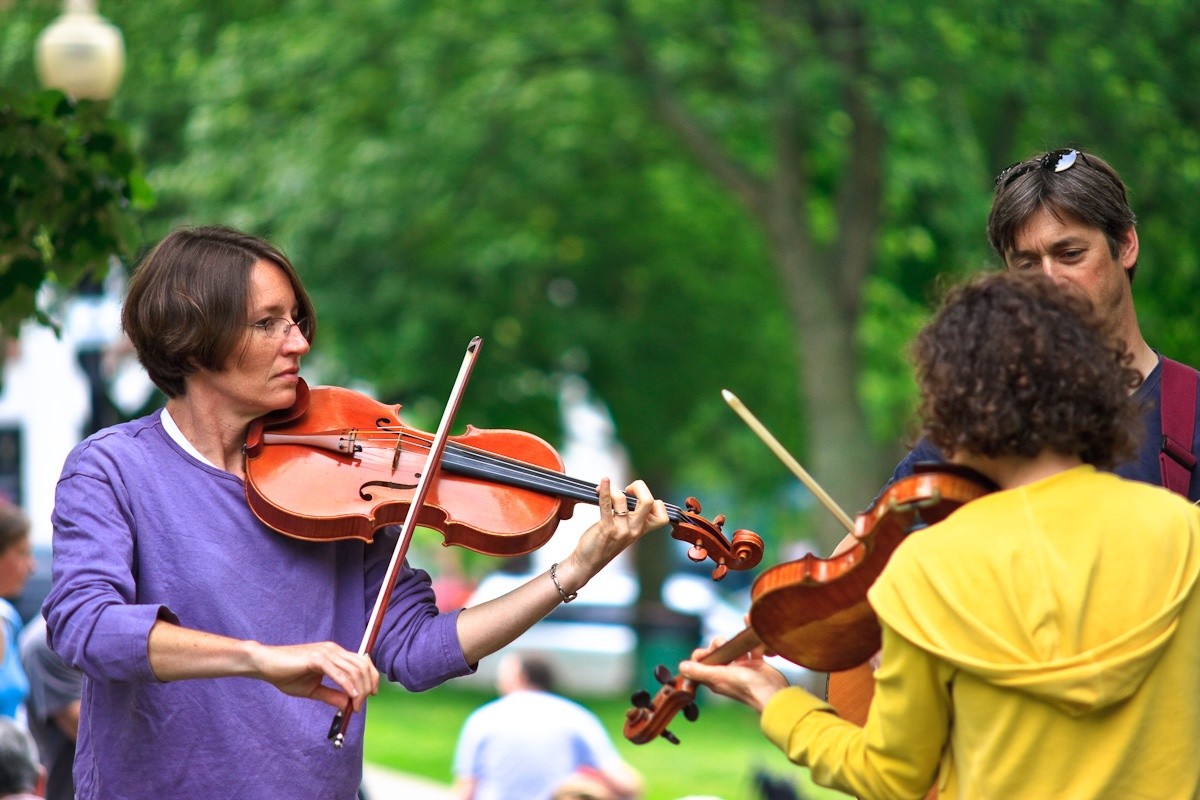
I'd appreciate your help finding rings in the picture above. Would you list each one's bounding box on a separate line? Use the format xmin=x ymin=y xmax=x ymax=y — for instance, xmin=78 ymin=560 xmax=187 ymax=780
xmin=613 ymin=509 xmax=630 ymax=516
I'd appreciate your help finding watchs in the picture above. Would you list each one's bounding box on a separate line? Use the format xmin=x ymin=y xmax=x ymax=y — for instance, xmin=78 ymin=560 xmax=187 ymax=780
xmin=551 ymin=563 xmax=577 ymax=603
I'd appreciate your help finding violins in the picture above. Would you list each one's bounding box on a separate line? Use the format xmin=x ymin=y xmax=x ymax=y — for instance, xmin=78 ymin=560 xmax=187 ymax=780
xmin=824 ymin=648 xmax=878 ymax=730
xmin=240 ymin=386 xmax=765 ymax=582
xmin=620 ymin=471 xmax=1003 ymax=747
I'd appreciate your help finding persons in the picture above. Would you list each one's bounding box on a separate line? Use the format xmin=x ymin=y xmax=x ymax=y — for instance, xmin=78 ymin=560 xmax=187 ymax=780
xmin=853 ymin=148 xmax=1200 ymax=505
xmin=0 ymin=498 xmax=83 ymax=800
xmin=679 ymin=266 xmax=1200 ymax=799
xmin=451 ymin=648 xmax=644 ymax=800
xmin=40 ymin=225 xmax=671 ymax=800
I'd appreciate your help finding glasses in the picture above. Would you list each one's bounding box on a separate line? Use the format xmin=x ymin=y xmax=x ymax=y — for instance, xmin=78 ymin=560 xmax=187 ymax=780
xmin=994 ymin=147 xmax=1093 ymax=187
xmin=245 ymin=318 xmax=312 ymax=339
